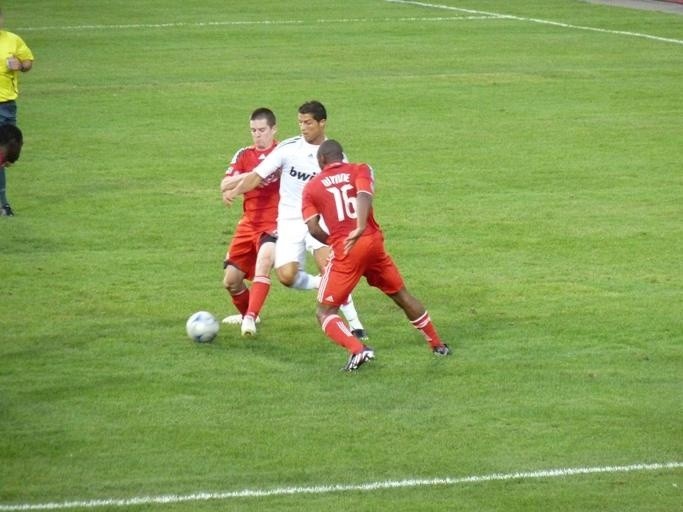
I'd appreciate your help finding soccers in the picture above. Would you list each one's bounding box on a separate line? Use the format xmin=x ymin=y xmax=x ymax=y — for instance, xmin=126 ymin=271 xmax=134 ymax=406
xmin=187 ymin=311 xmax=219 ymax=342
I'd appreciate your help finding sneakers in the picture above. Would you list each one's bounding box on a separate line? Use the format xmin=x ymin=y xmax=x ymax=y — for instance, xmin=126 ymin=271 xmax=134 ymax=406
xmin=431 ymin=343 xmax=452 ymax=359
xmin=221 ymin=313 xmax=261 ymax=325
xmin=0 ymin=203 xmax=14 ymax=217
xmin=337 ymin=344 xmax=375 ymax=374
xmin=341 ymin=329 xmax=369 ymax=347
xmin=239 ymin=315 xmax=256 ymax=337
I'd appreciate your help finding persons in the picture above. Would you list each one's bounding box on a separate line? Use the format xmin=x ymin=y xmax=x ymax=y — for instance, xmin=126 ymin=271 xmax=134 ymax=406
xmin=0 ymin=124 xmax=23 ymax=170
xmin=301 ymin=139 xmax=451 ymax=374
xmin=221 ymin=99 xmax=368 ymax=343
xmin=0 ymin=29 xmax=34 ymax=217
xmin=221 ymin=108 xmax=281 ymax=337
